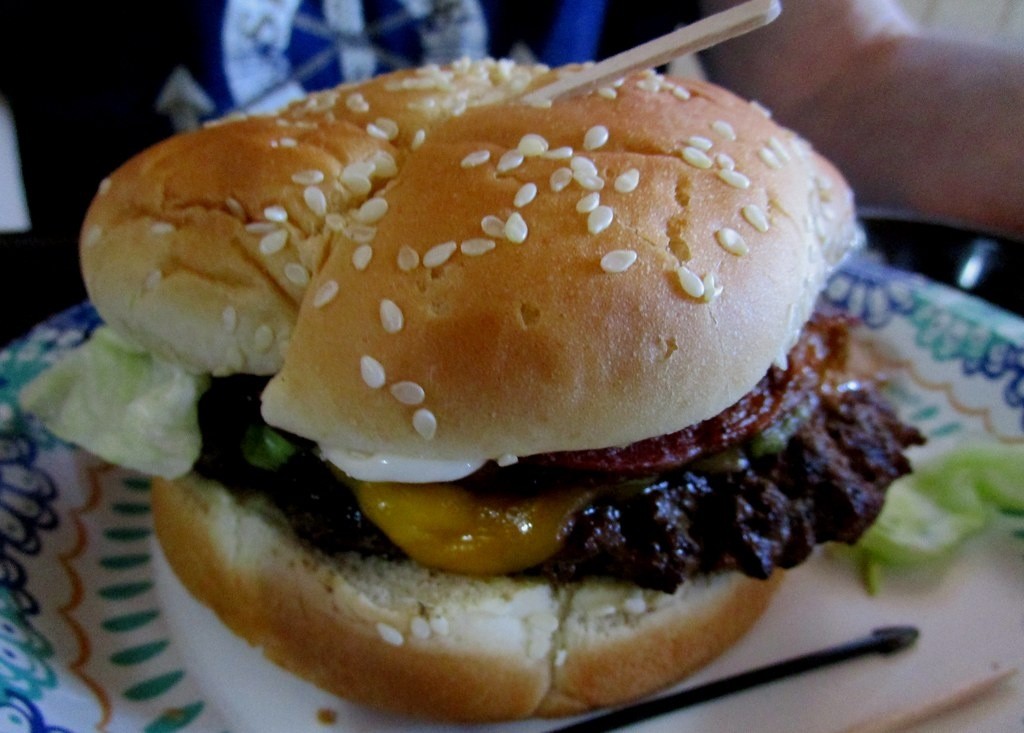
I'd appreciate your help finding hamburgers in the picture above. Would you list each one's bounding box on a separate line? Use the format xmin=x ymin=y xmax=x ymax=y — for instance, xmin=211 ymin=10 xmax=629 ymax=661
xmin=13 ymin=54 xmax=1024 ymax=725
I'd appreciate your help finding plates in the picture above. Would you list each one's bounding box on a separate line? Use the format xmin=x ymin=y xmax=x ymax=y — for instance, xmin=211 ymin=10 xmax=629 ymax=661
xmin=2 ymin=254 xmax=1024 ymax=733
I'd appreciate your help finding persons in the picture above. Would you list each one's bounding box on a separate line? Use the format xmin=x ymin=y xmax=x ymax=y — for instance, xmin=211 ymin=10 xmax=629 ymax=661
xmin=3 ymin=0 xmax=1023 ymax=343
